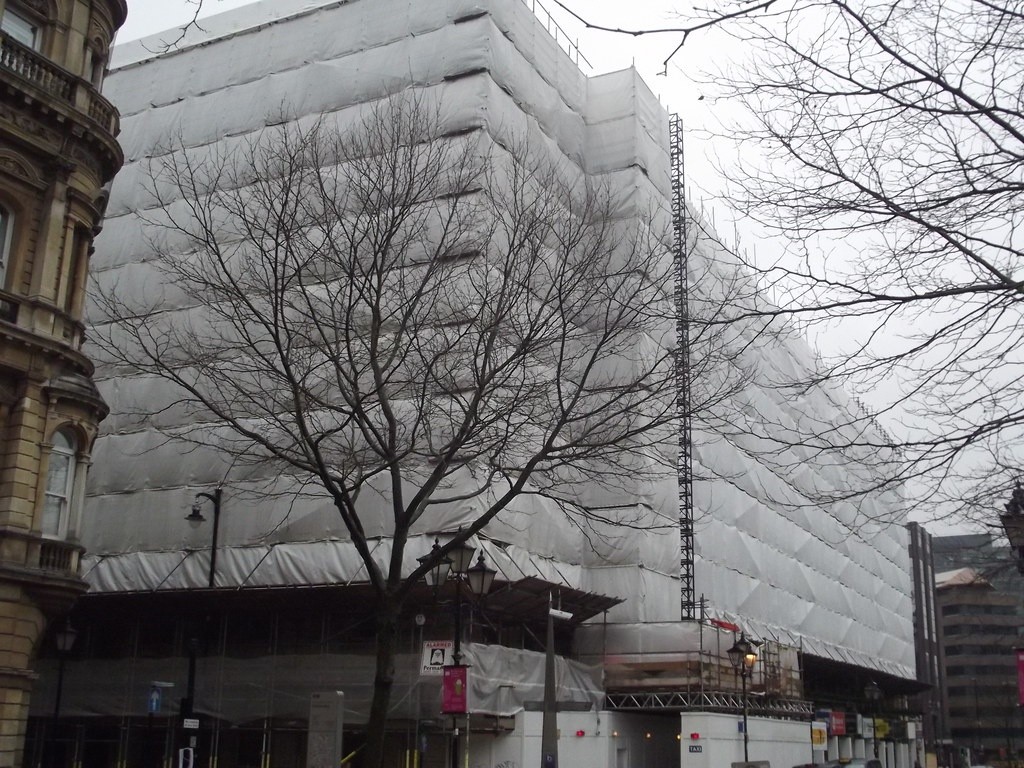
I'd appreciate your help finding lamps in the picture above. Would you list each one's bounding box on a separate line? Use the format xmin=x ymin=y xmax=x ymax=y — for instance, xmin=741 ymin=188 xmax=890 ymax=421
xmin=183 ymin=493 xmax=219 ymax=528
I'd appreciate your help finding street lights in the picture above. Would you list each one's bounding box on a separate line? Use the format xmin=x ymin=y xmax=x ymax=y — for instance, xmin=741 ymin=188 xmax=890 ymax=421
xmin=417 ymin=524 xmax=498 ymax=768
xmin=726 ymin=631 xmax=759 ymax=762
xmin=166 ymin=486 xmax=224 ymax=768
xmin=864 ymin=675 xmax=882 ymax=759
